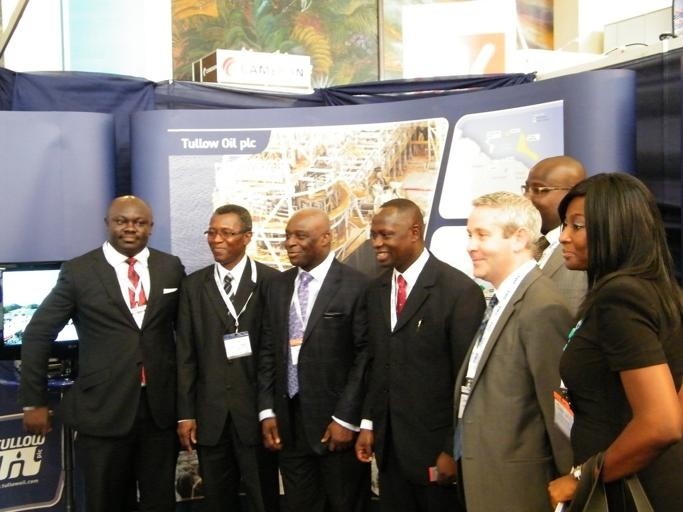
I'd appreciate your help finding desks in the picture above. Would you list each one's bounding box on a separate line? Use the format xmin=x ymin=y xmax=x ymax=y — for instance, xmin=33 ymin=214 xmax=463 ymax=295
xmin=0 ymin=361 xmax=82 ymax=510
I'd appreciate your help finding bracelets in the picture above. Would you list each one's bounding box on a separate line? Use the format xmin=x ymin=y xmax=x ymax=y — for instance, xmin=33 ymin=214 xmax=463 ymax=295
xmin=574 ymin=466 xmax=583 ymax=481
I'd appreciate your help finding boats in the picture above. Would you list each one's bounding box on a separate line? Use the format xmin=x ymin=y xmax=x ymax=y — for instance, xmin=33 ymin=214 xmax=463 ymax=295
xmin=213 ymin=120 xmax=438 ymax=273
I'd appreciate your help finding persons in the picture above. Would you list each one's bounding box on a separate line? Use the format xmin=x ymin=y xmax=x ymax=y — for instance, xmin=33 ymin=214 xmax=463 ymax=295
xmin=520 ymin=156 xmax=588 ymax=319
xmin=175 ymin=204 xmax=283 ymax=512
xmin=547 ymin=170 xmax=682 ymax=511
xmin=354 ymin=200 xmax=486 ymax=511
xmin=20 ymin=196 xmax=187 ymax=511
xmin=252 ymin=208 xmax=375 ymax=512
xmin=453 ymin=192 xmax=576 ymax=512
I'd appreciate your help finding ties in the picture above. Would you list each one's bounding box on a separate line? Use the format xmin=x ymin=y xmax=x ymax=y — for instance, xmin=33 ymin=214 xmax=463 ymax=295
xmin=470 ymin=294 xmax=501 ymax=352
xmin=126 ymin=256 xmax=147 ymax=386
xmin=396 ymin=275 xmax=408 ymax=321
xmin=222 ymin=275 xmax=238 ymax=322
xmin=286 ymin=271 xmax=313 ymax=399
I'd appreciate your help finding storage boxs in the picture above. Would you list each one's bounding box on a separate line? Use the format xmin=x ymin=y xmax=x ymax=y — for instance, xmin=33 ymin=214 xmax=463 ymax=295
xmin=188 ymin=47 xmax=314 ymax=94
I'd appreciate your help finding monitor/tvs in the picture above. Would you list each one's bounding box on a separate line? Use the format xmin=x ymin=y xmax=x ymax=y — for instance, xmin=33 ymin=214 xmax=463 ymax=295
xmin=0 ymin=260 xmax=79 ymax=360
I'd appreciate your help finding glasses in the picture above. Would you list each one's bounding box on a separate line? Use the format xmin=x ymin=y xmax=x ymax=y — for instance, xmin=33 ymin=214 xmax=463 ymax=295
xmin=522 ymin=183 xmax=572 ymax=198
xmin=204 ymin=226 xmax=244 ymax=239
